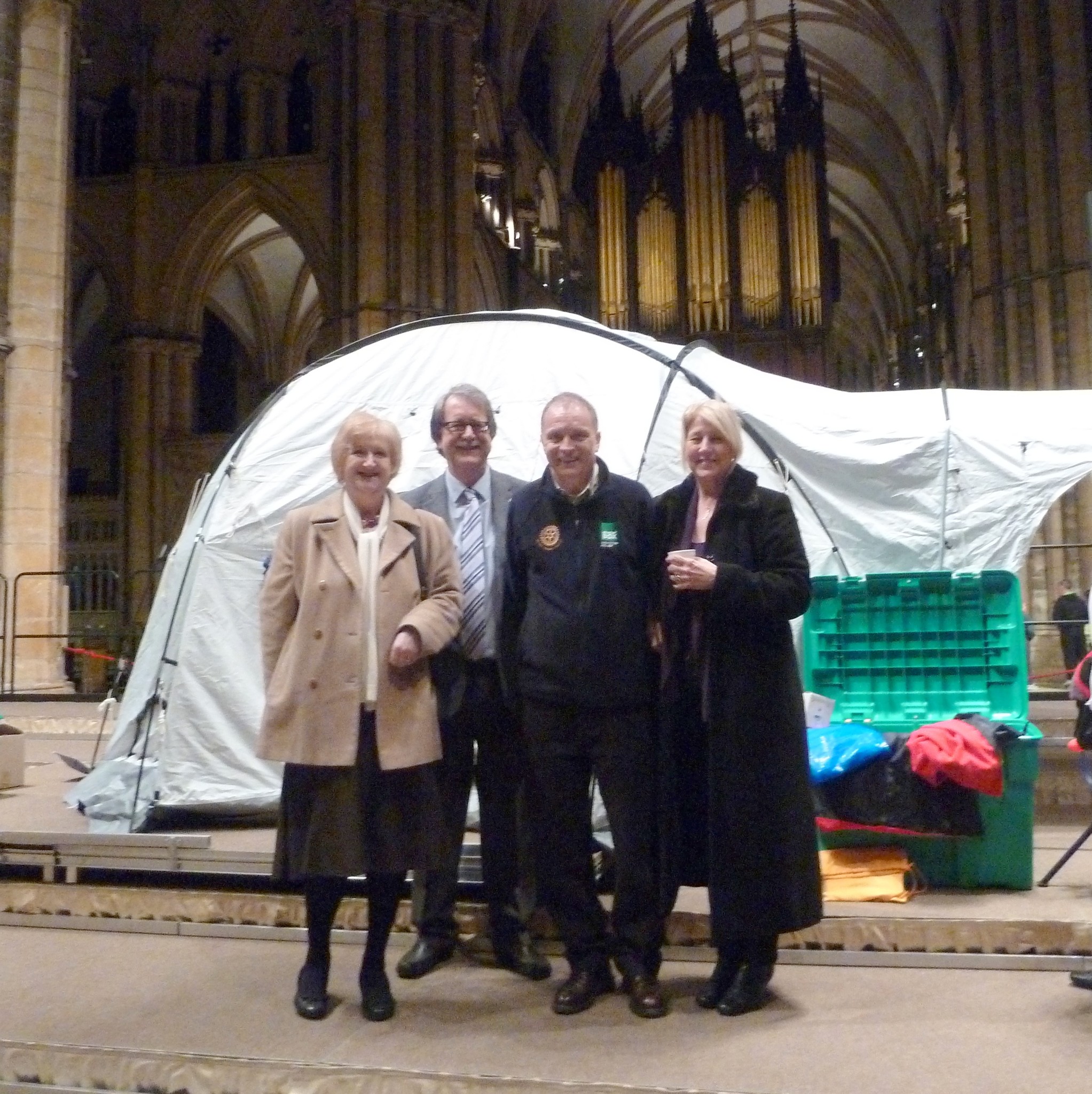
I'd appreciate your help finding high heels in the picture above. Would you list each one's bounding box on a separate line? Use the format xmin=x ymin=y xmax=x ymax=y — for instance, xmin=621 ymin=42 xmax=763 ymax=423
xmin=697 ymin=955 xmax=741 ymax=1008
xmin=719 ymin=960 xmax=774 ymax=1015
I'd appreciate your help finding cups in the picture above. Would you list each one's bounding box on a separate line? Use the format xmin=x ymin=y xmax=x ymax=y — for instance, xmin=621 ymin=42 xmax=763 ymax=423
xmin=667 ymin=549 xmax=696 ymax=585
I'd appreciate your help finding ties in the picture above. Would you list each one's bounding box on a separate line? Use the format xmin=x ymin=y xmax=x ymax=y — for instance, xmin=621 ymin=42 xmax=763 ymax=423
xmin=463 ymin=487 xmax=487 ymax=662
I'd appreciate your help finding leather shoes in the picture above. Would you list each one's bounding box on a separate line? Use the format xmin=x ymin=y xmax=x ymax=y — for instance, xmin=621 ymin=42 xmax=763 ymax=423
xmin=622 ymin=969 xmax=665 ymax=1019
xmin=396 ymin=931 xmax=454 ymax=977
xmin=295 ymin=957 xmax=328 ymax=1020
xmin=491 ymin=933 xmax=552 ymax=980
xmin=359 ymin=968 xmax=394 ymax=1023
xmin=553 ymin=964 xmax=612 ymax=1014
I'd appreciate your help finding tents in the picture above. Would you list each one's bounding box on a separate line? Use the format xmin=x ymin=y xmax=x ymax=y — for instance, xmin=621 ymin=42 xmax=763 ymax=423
xmin=104 ymin=310 xmax=1092 ymax=824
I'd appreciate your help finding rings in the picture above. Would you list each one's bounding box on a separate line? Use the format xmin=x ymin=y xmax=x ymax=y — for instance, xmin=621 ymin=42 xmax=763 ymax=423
xmin=676 ymin=575 xmax=681 ymax=582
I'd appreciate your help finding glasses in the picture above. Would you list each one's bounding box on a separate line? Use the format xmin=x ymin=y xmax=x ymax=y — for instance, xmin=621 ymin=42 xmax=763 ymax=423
xmin=442 ymin=421 xmax=491 ymax=433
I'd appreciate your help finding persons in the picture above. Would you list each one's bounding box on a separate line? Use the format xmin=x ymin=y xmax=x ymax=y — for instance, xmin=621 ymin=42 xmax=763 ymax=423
xmin=496 ymin=393 xmax=669 ymax=1017
xmin=1053 ymin=579 xmax=1089 ymax=685
xmin=1068 ymin=650 xmax=1092 ymax=787
xmin=396 ymin=383 xmax=552 ymax=979
xmin=650 ymin=399 xmax=823 ymax=1013
xmin=255 ymin=412 xmax=464 ymax=1023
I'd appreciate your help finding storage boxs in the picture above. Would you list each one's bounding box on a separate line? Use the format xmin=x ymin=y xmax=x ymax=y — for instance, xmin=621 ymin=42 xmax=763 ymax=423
xmin=794 ymin=567 xmax=1045 ymax=893
xmin=0 ymin=723 xmax=26 ymax=790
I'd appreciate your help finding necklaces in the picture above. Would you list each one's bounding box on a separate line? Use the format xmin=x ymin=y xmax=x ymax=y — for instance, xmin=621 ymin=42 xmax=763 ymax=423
xmin=701 ymin=509 xmax=709 ymax=520
